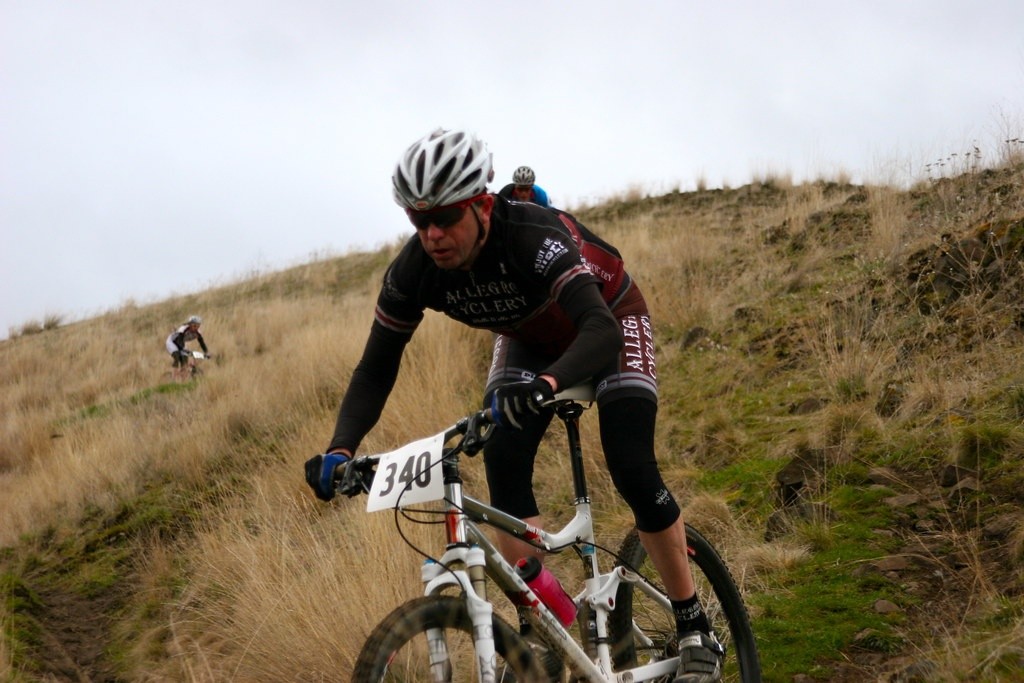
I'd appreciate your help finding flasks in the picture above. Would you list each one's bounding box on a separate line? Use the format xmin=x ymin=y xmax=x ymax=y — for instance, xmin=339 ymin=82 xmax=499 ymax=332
xmin=514 ymin=554 xmax=580 ymax=628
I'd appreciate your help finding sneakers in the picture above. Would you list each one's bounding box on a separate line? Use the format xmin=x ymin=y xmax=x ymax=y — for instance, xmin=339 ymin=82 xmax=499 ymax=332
xmin=672 ymin=629 xmax=726 ymax=683
xmin=499 ymin=641 xmax=559 ymax=683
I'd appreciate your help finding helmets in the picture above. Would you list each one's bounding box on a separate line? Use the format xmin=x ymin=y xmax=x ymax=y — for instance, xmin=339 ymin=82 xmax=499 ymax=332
xmin=391 ymin=126 xmax=494 ymax=210
xmin=512 ymin=165 xmax=535 ymax=185
xmin=189 ymin=316 xmax=201 ymax=323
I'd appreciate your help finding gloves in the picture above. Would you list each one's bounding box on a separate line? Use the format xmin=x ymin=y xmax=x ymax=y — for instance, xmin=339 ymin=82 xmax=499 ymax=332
xmin=303 ymin=451 xmax=350 ymax=501
xmin=493 ymin=378 xmax=556 ymax=431
xmin=205 ymin=353 xmax=211 ymax=359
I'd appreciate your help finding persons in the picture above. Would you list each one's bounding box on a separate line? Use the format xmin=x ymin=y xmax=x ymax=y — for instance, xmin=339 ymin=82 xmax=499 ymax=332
xmin=303 ymin=127 xmax=726 ymax=683
xmin=498 ymin=166 xmax=546 ymax=210
xmin=166 ymin=316 xmax=210 ymax=380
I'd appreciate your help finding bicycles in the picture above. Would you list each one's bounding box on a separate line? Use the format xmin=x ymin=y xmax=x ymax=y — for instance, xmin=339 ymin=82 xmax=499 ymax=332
xmin=158 ymin=349 xmax=211 ymax=386
xmin=328 ymin=380 xmax=763 ymax=683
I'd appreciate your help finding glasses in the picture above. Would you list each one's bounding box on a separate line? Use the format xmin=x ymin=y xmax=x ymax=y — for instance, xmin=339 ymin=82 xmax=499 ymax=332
xmin=516 ymin=187 xmax=531 ymax=192
xmin=406 ymin=196 xmax=486 ymax=230
